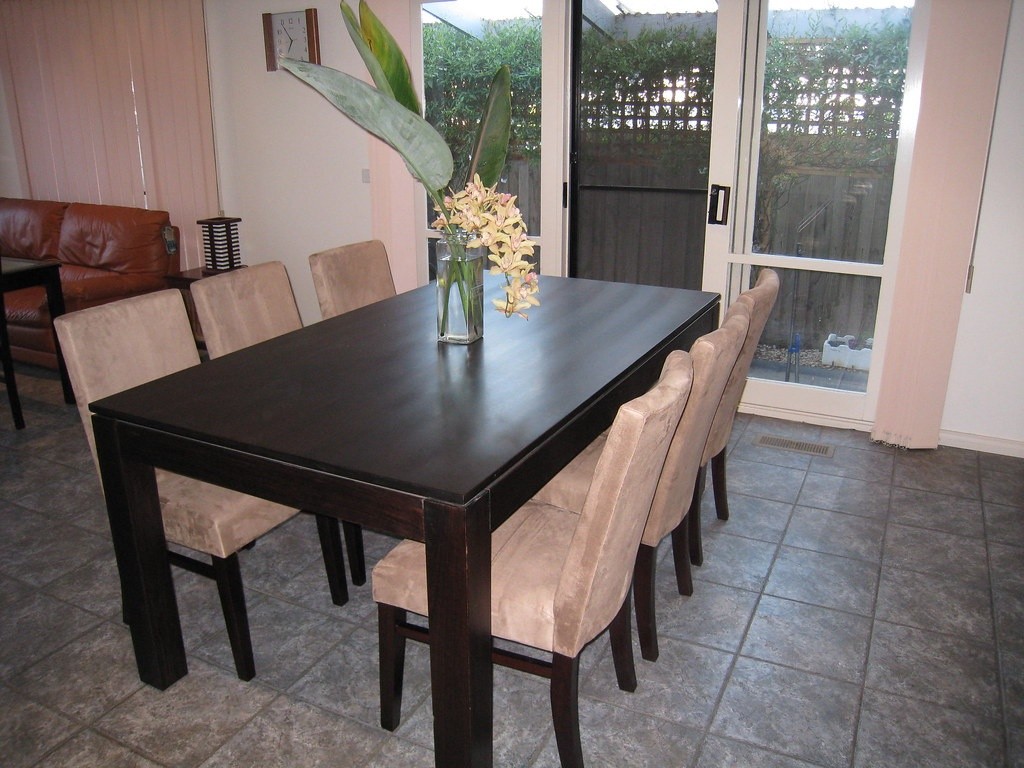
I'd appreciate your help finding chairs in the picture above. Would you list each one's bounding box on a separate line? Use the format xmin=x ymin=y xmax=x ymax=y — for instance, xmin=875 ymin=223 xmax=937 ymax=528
xmin=531 ymin=302 xmax=751 ymax=661
xmin=189 ymin=261 xmax=367 ymax=587
xmin=685 ymin=267 xmax=781 ymax=567
xmin=53 ymin=288 xmax=347 ymax=681
xmin=373 ymin=350 xmax=693 ymax=768
xmin=309 ymin=239 xmax=397 ymax=323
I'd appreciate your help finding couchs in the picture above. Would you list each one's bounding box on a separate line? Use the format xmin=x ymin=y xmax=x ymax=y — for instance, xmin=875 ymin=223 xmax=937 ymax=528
xmin=0 ymin=197 xmax=181 ymax=374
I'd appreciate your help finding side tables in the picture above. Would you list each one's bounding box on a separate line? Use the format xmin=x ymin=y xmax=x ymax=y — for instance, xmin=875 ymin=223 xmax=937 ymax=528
xmin=162 ymin=265 xmax=247 ymax=352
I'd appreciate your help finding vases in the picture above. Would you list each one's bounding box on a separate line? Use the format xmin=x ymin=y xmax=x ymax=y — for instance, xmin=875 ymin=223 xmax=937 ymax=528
xmin=434 ymin=230 xmax=485 ymax=343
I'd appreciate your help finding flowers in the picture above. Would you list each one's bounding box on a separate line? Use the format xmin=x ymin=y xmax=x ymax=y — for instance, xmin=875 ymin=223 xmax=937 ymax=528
xmin=431 ymin=175 xmax=540 ymax=322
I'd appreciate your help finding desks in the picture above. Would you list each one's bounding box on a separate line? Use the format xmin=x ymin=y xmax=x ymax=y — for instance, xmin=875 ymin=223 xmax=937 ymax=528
xmin=89 ymin=268 xmax=721 ymax=768
xmin=0 ymin=256 xmax=76 ymax=430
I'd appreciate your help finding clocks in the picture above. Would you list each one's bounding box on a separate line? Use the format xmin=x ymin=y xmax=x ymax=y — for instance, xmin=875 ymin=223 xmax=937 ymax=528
xmin=262 ymin=7 xmax=321 ymax=71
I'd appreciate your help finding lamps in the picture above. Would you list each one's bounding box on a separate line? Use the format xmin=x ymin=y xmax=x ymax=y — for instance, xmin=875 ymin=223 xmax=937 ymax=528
xmin=783 ymin=169 xmax=874 ymax=383
xmin=196 ymin=216 xmax=249 ymax=276
